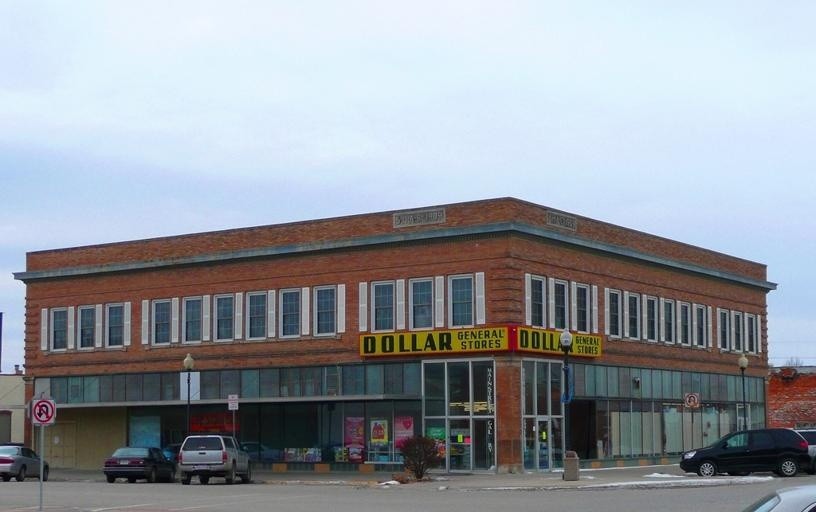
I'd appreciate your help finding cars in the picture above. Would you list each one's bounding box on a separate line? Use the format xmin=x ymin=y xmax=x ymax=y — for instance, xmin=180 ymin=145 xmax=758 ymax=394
xmin=680 ymin=428 xmax=816 ymax=477
xmin=741 ymin=485 xmax=816 ymax=512
xmin=239 ymin=442 xmax=283 ymax=461
xmin=0 ymin=442 xmax=49 ymax=482
xmin=177 ymin=436 xmax=252 ymax=484
xmin=103 ymin=447 xmax=176 ymax=483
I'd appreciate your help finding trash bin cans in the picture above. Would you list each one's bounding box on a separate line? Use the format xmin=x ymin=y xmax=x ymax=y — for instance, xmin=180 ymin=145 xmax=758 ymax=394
xmin=563 ymin=451 xmax=580 ymax=481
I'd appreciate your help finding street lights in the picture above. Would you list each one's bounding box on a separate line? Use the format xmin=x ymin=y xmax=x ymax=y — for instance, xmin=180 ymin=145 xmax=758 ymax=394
xmin=738 ymin=354 xmax=748 ymax=430
xmin=558 ymin=328 xmax=573 ymax=401
xmin=183 ymin=353 xmax=194 ymax=437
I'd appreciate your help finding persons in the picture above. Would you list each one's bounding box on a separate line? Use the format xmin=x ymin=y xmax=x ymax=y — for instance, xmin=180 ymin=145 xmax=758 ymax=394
xmin=437 ymin=440 xmax=446 ymax=468
